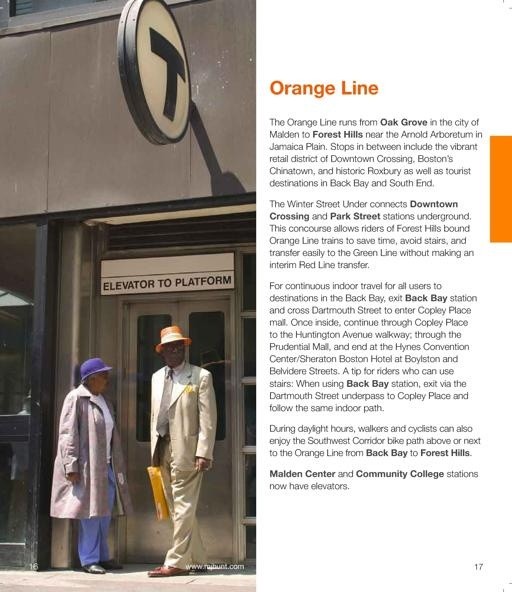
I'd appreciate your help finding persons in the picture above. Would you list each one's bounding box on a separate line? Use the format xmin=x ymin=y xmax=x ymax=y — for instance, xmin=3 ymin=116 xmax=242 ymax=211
xmin=50 ymin=358 xmax=137 ymax=573
xmin=146 ymin=325 xmax=217 ymax=577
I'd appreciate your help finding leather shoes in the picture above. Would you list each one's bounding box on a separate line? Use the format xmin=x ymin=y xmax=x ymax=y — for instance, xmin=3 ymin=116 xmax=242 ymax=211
xmin=190 ymin=566 xmax=209 ymax=573
xmin=82 ymin=563 xmax=107 ymax=575
xmin=100 ymin=559 xmax=124 ymax=570
xmin=147 ymin=564 xmax=191 ymax=577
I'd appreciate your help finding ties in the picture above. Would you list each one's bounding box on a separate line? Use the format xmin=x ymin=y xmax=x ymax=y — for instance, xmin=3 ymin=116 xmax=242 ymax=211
xmin=157 ymin=368 xmax=175 ymax=438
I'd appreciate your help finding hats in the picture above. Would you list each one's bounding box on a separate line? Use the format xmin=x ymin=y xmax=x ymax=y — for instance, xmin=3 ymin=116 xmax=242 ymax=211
xmin=154 ymin=324 xmax=192 ymax=354
xmin=79 ymin=356 xmax=114 ymax=382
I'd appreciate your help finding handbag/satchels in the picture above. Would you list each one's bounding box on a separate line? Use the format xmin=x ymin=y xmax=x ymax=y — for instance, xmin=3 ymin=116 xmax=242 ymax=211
xmin=146 ymin=465 xmax=171 ymax=523
